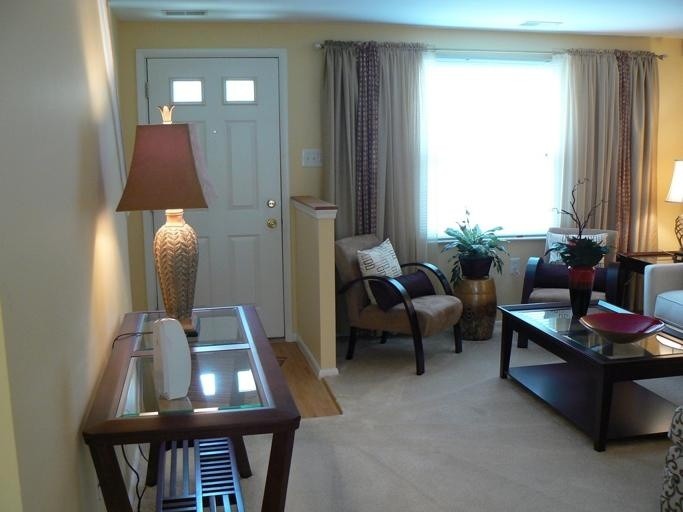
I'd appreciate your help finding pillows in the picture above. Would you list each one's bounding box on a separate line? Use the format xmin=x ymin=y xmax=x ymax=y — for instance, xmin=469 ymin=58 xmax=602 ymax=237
xmin=534 ymin=265 xmax=608 ymax=292
xmin=547 ymin=233 xmax=608 ymax=269
xmin=368 ymin=269 xmax=436 ymax=312
xmin=356 ymin=238 xmax=404 ymax=306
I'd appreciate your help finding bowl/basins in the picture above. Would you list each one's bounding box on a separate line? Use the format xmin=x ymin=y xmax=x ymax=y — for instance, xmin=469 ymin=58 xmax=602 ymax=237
xmin=580 ymin=312 xmax=666 ymax=345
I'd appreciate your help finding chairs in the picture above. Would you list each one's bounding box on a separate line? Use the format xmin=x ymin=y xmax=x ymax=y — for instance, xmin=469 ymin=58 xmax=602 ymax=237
xmin=335 ymin=233 xmax=463 ymax=375
xmin=517 ymin=226 xmax=627 ymax=348
xmin=643 ymin=262 xmax=683 ymax=334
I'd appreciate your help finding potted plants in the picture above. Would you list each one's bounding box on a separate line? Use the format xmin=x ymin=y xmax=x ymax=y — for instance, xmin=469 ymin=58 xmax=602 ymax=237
xmin=440 ymin=219 xmax=511 ymax=290
xmin=543 ymin=177 xmax=611 ymax=318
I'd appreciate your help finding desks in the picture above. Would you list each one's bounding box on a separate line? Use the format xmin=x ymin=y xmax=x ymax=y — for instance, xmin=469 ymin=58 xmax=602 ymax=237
xmin=83 ymin=303 xmax=301 ymax=512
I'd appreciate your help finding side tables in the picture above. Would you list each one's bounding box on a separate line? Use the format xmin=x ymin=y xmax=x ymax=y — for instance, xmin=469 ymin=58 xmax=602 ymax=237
xmin=616 ymin=250 xmax=683 ymax=315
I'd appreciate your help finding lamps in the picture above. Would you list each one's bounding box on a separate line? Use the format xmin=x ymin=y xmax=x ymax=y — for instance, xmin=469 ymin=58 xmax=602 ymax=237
xmin=115 ymin=104 xmax=209 ymax=338
xmin=663 ymin=160 xmax=683 ymax=262
